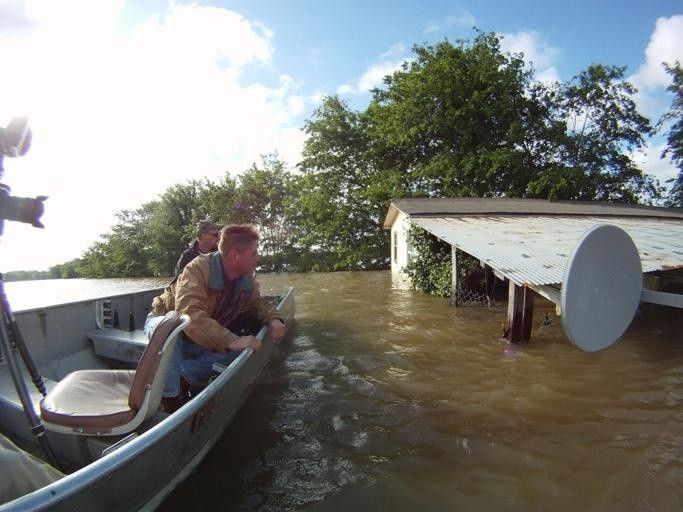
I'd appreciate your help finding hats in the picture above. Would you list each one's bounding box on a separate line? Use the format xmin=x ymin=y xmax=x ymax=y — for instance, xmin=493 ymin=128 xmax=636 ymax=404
xmin=197 ymin=220 xmax=224 ymax=234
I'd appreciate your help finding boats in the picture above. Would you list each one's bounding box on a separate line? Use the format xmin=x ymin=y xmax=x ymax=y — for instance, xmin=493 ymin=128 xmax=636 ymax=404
xmin=0 ymin=281 xmax=298 ymax=512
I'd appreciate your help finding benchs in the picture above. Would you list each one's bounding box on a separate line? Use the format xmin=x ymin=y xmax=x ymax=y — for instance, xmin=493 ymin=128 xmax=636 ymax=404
xmin=85 ymin=326 xmax=151 ymax=364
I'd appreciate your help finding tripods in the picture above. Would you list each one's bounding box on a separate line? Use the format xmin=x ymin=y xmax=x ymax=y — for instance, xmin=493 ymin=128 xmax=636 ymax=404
xmin=0 ymin=275 xmax=62 ymax=470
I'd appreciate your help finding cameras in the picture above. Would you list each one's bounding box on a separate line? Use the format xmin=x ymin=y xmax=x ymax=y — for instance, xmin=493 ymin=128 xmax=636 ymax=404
xmin=0 ymin=117 xmax=47 ymax=232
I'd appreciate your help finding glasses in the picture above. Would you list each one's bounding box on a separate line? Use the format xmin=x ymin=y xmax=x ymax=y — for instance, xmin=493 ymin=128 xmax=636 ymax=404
xmin=208 ymin=232 xmax=218 ymax=237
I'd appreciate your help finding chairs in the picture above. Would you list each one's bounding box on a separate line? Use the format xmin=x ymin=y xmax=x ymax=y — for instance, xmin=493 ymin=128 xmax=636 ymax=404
xmin=40 ymin=309 xmax=193 ymax=438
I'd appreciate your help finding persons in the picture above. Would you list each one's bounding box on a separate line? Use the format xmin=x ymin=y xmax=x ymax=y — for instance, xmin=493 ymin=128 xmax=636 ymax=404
xmin=143 ymin=224 xmax=287 ymax=414
xmin=173 ymin=220 xmax=223 ymax=278
